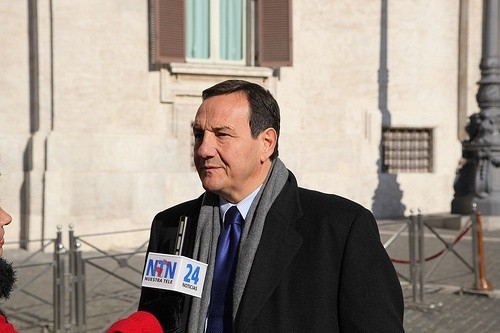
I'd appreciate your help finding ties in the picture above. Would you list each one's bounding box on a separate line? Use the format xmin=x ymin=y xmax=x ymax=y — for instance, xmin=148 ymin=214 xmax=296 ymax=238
xmin=205 ymin=206 xmax=245 ymax=333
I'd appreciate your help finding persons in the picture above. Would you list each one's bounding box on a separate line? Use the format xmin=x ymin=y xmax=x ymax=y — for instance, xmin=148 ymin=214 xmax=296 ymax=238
xmin=0 ymin=205 xmax=186 ymax=333
xmin=136 ymin=79 xmax=405 ymax=333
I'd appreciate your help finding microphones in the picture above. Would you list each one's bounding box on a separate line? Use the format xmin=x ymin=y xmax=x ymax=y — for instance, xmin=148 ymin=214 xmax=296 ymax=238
xmin=142 ymin=216 xmax=209 ymax=299
